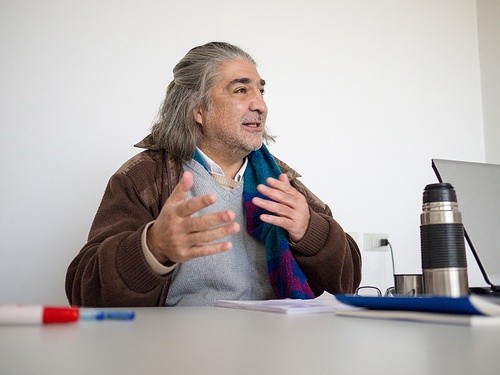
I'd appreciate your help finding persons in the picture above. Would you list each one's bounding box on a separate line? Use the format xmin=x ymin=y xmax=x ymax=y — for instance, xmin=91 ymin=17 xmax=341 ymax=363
xmin=65 ymin=41 xmax=362 ymax=308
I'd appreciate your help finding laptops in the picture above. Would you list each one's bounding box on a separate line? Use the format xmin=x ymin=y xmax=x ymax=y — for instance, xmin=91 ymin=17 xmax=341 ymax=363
xmin=431 ymin=157 xmax=500 ymax=304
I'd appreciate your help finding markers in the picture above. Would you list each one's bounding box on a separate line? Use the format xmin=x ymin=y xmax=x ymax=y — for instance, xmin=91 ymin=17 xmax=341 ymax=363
xmin=0 ymin=305 xmax=80 ymax=326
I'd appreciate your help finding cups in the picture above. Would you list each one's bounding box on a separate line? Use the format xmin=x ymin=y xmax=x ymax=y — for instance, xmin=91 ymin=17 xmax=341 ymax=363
xmin=393 ymin=272 xmax=423 ymax=296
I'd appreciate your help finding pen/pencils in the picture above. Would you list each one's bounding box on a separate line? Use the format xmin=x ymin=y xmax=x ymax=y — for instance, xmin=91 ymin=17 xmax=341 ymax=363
xmin=79 ymin=308 xmax=136 ymax=322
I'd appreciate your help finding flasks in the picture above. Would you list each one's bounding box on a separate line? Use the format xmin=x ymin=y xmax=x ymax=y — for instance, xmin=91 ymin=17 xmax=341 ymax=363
xmin=418 ymin=182 xmax=468 ymax=297
